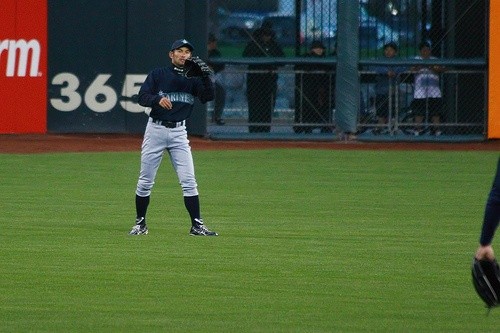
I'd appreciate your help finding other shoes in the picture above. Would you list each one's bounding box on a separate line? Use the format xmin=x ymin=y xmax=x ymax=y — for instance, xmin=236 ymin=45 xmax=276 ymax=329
xmin=215 ymin=117 xmax=224 ymax=125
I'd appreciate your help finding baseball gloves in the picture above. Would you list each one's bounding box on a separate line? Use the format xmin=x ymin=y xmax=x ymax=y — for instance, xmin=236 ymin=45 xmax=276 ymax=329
xmin=470 ymin=256 xmax=500 ymax=308
xmin=182 ymin=56 xmax=212 ymax=80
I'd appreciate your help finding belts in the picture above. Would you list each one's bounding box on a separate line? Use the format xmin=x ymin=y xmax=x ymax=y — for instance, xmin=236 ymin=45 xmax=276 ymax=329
xmin=152 ymin=118 xmax=183 ymax=127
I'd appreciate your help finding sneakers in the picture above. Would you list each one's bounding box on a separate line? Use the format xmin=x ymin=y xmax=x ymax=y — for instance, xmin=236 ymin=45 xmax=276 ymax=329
xmin=128 ymin=224 xmax=149 ymax=236
xmin=188 ymin=225 xmax=219 ymax=237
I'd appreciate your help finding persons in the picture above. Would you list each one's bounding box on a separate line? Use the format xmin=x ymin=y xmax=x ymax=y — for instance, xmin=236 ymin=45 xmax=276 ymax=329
xmin=128 ymin=39 xmax=217 ymax=236
xmin=410 ymin=40 xmax=448 ymax=136
xmin=374 ymin=42 xmax=408 ymax=132
xmin=243 ymin=22 xmax=287 ymax=133
xmin=474 ymin=156 xmax=500 ymax=260
xmin=293 ymin=41 xmax=338 ymax=134
xmin=207 ymin=31 xmax=225 ymax=126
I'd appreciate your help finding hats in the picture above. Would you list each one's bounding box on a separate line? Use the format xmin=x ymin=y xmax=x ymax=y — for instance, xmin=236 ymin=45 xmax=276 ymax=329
xmin=310 ymin=41 xmax=326 ymax=49
xmin=169 ymin=39 xmax=193 ymax=52
xmin=384 ymin=41 xmax=398 ymax=49
